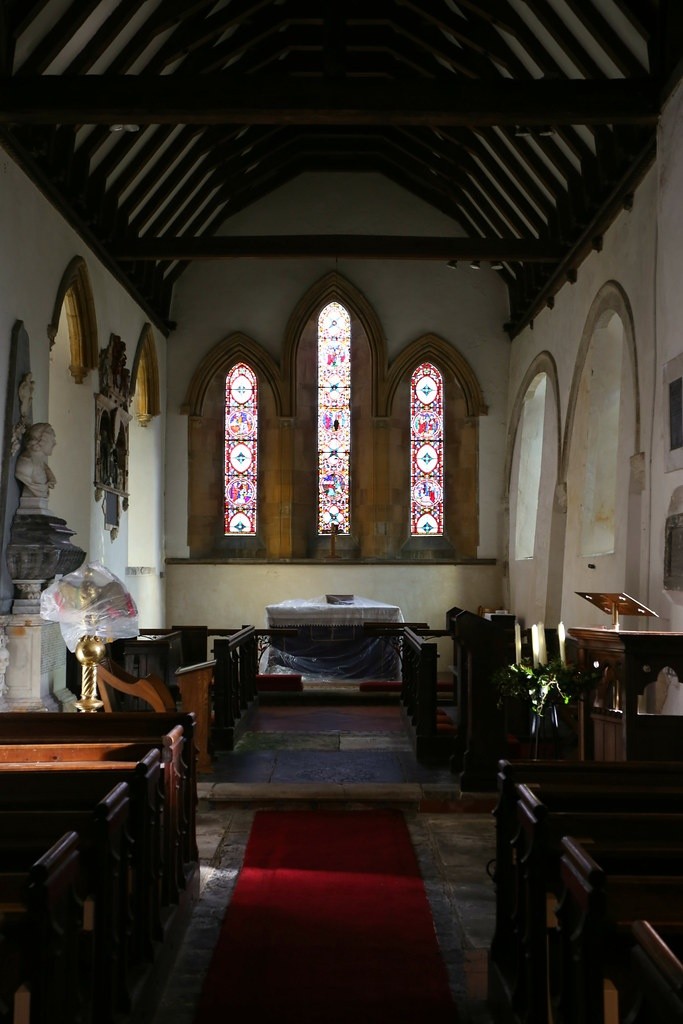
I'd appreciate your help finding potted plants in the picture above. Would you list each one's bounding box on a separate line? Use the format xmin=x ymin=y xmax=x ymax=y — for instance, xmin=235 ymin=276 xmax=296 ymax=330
xmin=493 ymin=655 xmax=593 ymax=720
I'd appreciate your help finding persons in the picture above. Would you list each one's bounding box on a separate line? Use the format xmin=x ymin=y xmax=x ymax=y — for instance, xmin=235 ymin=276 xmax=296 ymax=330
xmin=15 ymin=422 xmax=57 ymax=516
xmin=19 ymin=372 xmax=35 ymax=416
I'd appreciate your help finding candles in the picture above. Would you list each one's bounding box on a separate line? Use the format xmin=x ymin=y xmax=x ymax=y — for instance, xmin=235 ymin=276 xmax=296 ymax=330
xmin=515 ymin=621 xmax=567 ymax=669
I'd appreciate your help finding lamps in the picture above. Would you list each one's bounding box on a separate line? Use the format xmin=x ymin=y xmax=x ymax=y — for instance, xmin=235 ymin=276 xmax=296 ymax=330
xmin=516 ymin=123 xmax=556 ymax=136
xmin=445 ymin=259 xmax=504 ymax=270
xmin=107 ymin=124 xmax=141 ymax=132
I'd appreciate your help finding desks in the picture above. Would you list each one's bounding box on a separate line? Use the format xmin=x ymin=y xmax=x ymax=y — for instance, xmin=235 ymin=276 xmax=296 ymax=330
xmin=266 ymin=598 xmax=402 ymax=681
xmin=485 ymin=612 xmax=517 ymax=629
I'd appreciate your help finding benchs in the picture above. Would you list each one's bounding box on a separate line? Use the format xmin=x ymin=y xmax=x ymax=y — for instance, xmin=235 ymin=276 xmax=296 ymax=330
xmin=0 ymin=710 xmax=198 ymax=1024
xmin=482 ymin=761 xmax=683 ymax=1024
